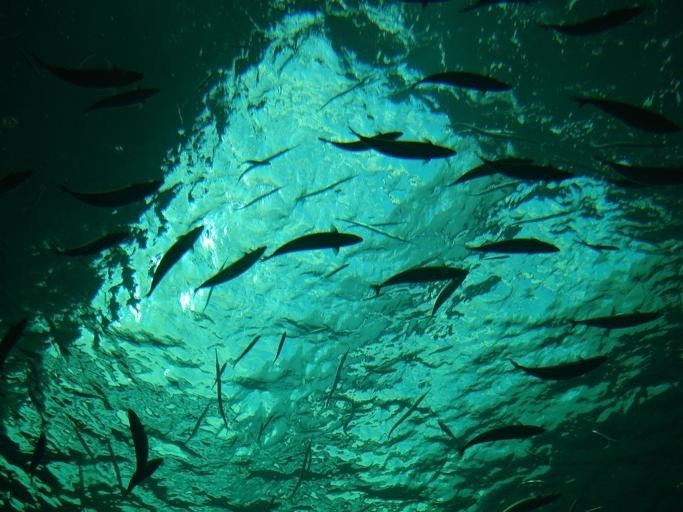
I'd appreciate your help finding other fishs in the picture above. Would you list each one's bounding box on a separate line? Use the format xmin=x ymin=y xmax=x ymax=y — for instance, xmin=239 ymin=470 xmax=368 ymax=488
xmin=0 ymin=1 xmax=682 ymax=510
